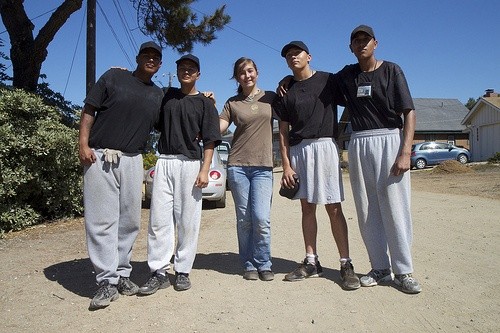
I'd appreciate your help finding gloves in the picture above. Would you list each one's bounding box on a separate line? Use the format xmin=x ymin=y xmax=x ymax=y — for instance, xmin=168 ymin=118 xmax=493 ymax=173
xmin=103 ymin=148 xmax=123 ymax=164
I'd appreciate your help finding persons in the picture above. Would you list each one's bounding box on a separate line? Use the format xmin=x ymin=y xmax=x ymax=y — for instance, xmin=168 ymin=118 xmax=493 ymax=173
xmin=279 ymin=41 xmax=361 ymax=291
xmin=78 ymin=41 xmax=166 ymax=310
xmin=200 ymin=57 xmax=276 ymax=281
xmin=278 ymin=25 xmax=423 ymax=294
xmin=110 ymin=54 xmax=223 ymax=296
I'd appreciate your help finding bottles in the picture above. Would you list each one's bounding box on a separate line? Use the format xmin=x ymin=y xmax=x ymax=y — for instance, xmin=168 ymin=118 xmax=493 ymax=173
xmin=279 ymin=174 xmax=300 ymax=199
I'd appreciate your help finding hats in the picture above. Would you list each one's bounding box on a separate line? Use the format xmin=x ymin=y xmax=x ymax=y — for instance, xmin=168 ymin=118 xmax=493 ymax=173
xmin=176 ymin=54 xmax=200 ymax=72
xmin=281 ymin=40 xmax=310 ymax=57
xmin=139 ymin=41 xmax=162 ymax=59
xmin=351 ymin=25 xmax=375 ymax=42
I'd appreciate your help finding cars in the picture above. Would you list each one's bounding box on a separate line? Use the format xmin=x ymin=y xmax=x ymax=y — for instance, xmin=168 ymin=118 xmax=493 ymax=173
xmin=142 ymin=138 xmax=229 ymax=209
xmin=410 ymin=141 xmax=470 ymax=170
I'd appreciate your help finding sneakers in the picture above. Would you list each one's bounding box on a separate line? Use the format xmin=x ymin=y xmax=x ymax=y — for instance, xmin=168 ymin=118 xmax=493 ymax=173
xmin=90 ymin=279 xmax=118 ymax=308
xmin=138 ymin=271 xmax=171 ymax=294
xmin=359 ymin=268 xmax=393 ymax=287
xmin=174 ymin=272 xmax=191 ymax=291
xmin=393 ymin=273 xmax=421 ymax=293
xmin=259 ymin=270 xmax=274 ymax=280
xmin=285 ymin=258 xmax=323 ymax=281
xmin=243 ymin=269 xmax=259 ymax=280
xmin=339 ymin=262 xmax=361 ymax=289
xmin=116 ymin=277 xmax=140 ymax=296
